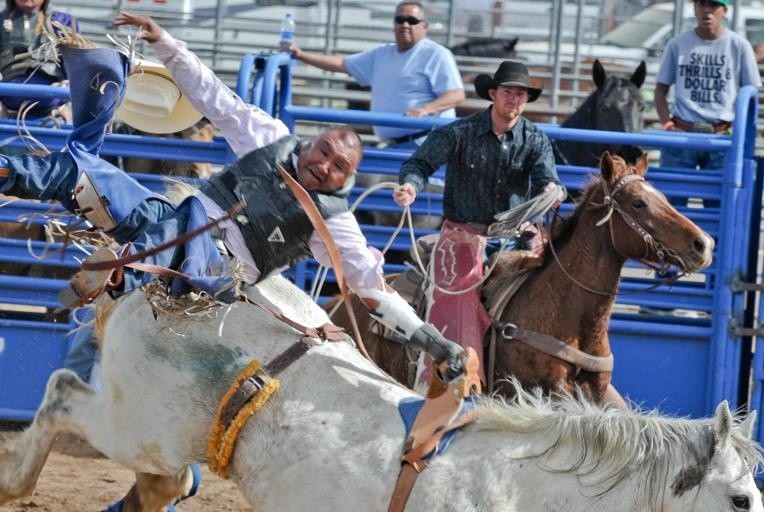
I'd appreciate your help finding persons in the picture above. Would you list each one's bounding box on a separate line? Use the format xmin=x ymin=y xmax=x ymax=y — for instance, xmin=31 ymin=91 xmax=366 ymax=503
xmin=0 ymin=10 xmax=465 ymax=387
xmin=282 ymin=0 xmax=466 ymax=180
xmin=638 ymin=0 xmax=763 ymax=316
xmin=393 ymin=62 xmax=567 ymax=394
xmin=0 ymin=0 xmax=80 ymax=125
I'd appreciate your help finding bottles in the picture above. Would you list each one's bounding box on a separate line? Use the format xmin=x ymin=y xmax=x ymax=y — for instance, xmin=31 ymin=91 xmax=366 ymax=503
xmin=279 ymin=13 xmax=296 ymax=55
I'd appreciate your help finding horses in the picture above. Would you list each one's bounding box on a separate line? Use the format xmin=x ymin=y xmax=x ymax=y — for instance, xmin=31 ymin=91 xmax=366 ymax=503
xmin=0 ymin=108 xmax=213 ymax=322
xmin=320 ymin=147 xmax=716 ymax=410
xmin=0 ymin=269 xmax=764 ymax=511
xmin=345 ymin=33 xmax=520 ymax=109
xmin=348 ymin=58 xmax=648 ymax=265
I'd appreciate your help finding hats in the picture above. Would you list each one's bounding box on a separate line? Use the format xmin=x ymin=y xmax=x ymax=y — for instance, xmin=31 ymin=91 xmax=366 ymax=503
xmin=474 ymin=61 xmax=545 ymax=105
xmin=714 ymin=0 xmax=729 ymax=8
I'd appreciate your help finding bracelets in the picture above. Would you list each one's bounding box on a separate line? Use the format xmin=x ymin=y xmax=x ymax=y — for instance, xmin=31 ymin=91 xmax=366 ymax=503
xmin=662 ymin=121 xmax=675 ymax=131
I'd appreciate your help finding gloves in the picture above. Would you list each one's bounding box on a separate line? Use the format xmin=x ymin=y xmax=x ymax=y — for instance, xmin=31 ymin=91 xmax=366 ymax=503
xmin=410 ymin=323 xmax=466 ymax=385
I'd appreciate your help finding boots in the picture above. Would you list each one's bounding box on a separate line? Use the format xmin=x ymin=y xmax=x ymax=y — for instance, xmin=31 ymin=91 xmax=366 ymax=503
xmin=60 ymin=248 xmax=119 ymax=307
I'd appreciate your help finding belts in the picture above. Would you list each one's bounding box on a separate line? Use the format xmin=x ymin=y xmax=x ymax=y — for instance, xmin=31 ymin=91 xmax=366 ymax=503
xmin=671 ymin=116 xmax=731 ymax=134
xmin=392 ymin=129 xmax=430 ymax=143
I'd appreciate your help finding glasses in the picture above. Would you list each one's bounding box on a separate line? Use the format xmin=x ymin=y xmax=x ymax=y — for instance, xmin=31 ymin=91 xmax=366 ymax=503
xmin=395 ymin=16 xmax=422 ymax=25
